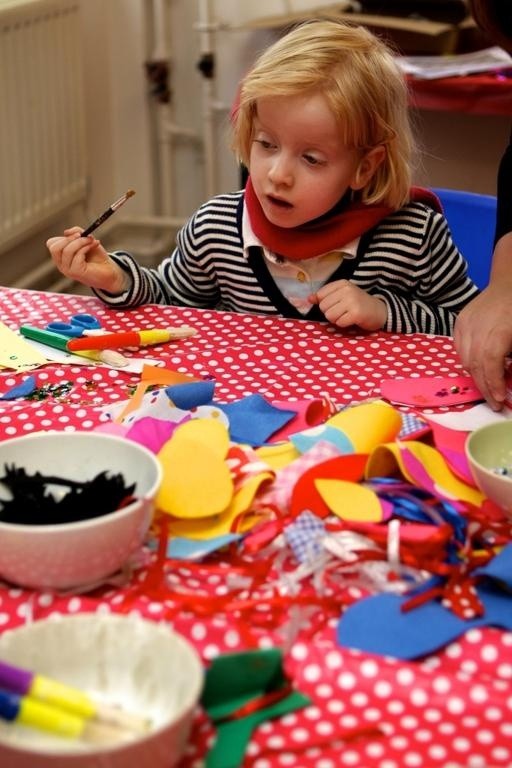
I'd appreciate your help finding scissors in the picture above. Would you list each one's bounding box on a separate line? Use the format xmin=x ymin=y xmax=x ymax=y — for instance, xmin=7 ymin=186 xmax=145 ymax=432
xmin=47 ymin=314 xmax=140 ymax=351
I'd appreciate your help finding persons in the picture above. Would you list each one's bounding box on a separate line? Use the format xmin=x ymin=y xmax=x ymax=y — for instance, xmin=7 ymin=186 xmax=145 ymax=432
xmin=43 ymin=19 xmax=483 ymax=337
xmin=449 ymin=143 xmax=512 ymax=417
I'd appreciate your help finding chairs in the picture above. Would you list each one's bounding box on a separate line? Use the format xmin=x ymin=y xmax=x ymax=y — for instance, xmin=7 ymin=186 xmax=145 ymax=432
xmin=428 ymin=186 xmax=500 ymax=292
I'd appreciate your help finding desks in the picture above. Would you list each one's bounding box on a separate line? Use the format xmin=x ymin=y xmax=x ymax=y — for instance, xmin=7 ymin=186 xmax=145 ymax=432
xmin=0 ymin=286 xmax=512 ymax=768
xmin=234 ymin=65 xmax=512 ymax=189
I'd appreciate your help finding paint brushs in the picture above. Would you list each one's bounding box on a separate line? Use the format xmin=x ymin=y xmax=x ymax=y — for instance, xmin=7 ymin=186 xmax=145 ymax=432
xmin=20 ymin=324 xmax=129 ymax=367
xmin=81 ymin=190 xmax=136 ymax=237
xmin=0 ymin=656 xmax=153 ymax=748
xmin=67 ymin=327 xmax=199 ymax=351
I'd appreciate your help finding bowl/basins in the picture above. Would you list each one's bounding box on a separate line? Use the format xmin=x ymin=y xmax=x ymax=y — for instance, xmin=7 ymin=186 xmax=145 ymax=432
xmin=1 ymin=430 xmax=163 ymax=591
xmin=466 ymin=416 xmax=512 ymax=522
xmin=1 ymin=614 xmax=205 ymax=762
